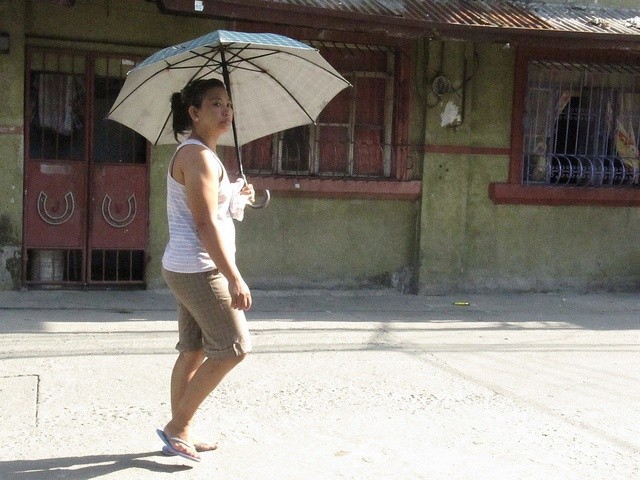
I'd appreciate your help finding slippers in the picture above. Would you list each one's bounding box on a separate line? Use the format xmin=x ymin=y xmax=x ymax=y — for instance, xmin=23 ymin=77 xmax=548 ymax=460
xmin=163 ymin=443 xmax=219 ymax=456
xmin=155 ymin=429 xmax=203 ymax=463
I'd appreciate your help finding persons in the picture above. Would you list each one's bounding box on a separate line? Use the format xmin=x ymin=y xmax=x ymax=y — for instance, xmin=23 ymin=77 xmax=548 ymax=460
xmin=156 ymin=77 xmax=256 ymax=462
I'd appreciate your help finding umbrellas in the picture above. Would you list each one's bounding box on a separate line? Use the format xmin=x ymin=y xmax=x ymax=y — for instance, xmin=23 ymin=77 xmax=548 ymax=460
xmin=106 ymin=30 xmax=354 ymax=209
